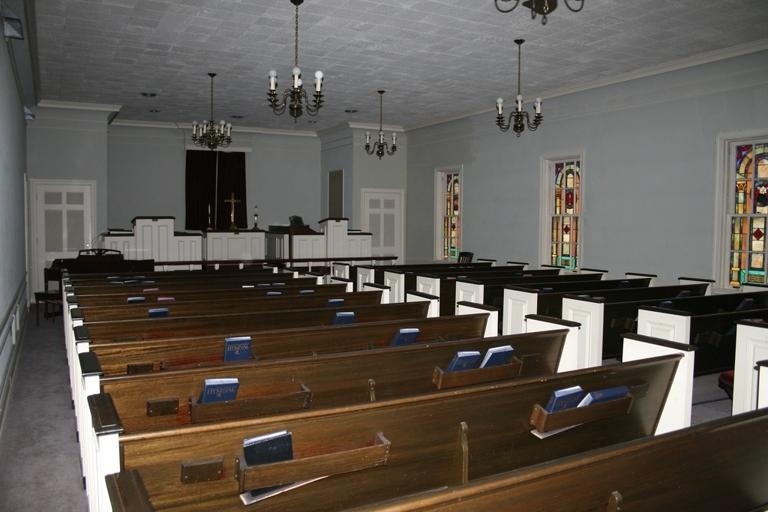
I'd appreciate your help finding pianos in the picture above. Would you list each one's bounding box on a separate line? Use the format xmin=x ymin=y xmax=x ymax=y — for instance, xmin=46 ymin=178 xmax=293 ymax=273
xmin=45 ymin=249 xmax=154 ymax=320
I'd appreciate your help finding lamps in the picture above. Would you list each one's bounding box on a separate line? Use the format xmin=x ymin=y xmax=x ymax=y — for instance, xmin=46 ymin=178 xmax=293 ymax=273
xmin=497 ymin=38 xmax=544 ymax=134
xmin=268 ymin=0 xmax=324 ymax=116
xmin=192 ymin=72 xmax=232 ymax=150
xmin=364 ymin=90 xmax=398 ymax=158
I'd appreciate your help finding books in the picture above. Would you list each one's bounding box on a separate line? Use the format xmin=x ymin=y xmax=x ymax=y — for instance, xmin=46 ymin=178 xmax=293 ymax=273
xmin=546 ymin=386 xmax=628 ymax=413
xmin=336 ymin=312 xmax=354 ymax=325
xmin=202 ymin=378 xmax=240 ymax=403
xmin=242 ymin=283 xmax=315 ymax=296
xmin=224 ymin=336 xmax=251 ymax=361
xmin=244 ymin=430 xmax=294 ymax=497
xmin=328 ymin=299 xmax=344 ymax=307
xmin=395 ymin=328 xmax=420 ymax=344
xmin=448 ymin=344 xmax=515 ymax=372
xmin=107 ymin=276 xmax=175 ymax=317
